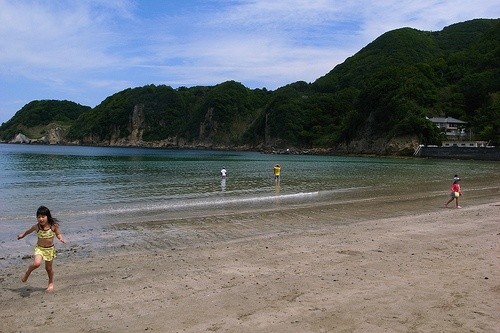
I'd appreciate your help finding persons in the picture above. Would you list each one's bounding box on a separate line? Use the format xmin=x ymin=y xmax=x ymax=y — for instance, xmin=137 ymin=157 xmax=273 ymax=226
xmin=17 ymin=206 xmax=64 ymax=290
xmin=220 ymin=166 xmax=227 ymax=177
xmin=449 ymin=174 xmax=458 ymax=184
xmin=445 ymin=177 xmax=462 ymax=209
xmin=274 ymin=164 xmax=281 ymax=183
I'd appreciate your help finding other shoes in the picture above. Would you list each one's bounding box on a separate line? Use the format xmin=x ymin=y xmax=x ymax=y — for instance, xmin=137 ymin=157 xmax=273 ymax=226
xmin=445 ymin=204 xmax=449 ymax=208
xmin=456 ymin=206 xmax=462 ymax=209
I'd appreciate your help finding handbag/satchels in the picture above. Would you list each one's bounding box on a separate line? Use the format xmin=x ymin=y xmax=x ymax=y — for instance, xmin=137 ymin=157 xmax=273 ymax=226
xmin=453 ymin=192 xmax=460 ymax=197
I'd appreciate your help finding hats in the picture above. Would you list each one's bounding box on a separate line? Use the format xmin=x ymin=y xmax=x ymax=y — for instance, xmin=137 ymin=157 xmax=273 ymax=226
xmin=450 ymin=177 xmax=461 ymax=183
